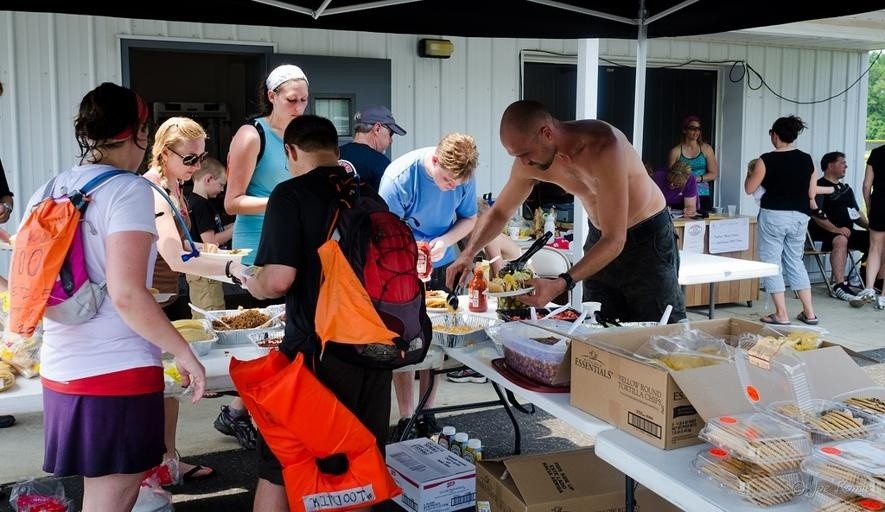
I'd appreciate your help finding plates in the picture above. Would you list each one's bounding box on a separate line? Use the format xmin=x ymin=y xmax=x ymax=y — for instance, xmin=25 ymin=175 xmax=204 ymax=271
xmin=240 ymin=265 xmax=263 ymax=281
xmin=482 ymin=276 xmax=540 ymax=298
xmin=200 ymin=248 xmax=253 ymax=257
xmin=492 ymin=358 xmax=570 ymax=394
xmin=426 ymin=307 xmax=448 ymax=313
xmin=507 ymin=235 xmax=532 ymax=243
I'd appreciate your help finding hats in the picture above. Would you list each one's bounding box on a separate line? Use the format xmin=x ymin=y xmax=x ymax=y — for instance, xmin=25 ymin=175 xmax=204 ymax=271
xmin=354 ymin=104 xmax=407 ymax=136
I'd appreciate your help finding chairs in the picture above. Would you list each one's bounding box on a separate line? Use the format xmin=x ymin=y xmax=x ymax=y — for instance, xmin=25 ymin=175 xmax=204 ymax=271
xmin=804 ymin=230 xmax=865 ymax=298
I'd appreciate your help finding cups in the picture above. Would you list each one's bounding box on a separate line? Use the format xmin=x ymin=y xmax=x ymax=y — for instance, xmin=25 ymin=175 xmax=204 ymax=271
xmin=814 ymin=241 xmax=823 ymax=252
xmin=712 ymin=206 xmax=721 ymax=215
xmin=582 ymin=301 xmax=602 ymax=325
xmin=507 ymin=226 xmax=520 ymax=241
xmin=727 ymin=204 xmax=737 ymax=218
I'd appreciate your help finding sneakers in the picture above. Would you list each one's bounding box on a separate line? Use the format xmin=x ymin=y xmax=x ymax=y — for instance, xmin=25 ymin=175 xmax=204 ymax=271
xmin=412 ymin=412 xmax=437 ymax=437
xmin=0 ymin=415 xmax=15 ymax=428
xmin=874 ymin=281 xmax=884 ymax=294
xmin=877 ymin=296 xmax=885 ymax=309
xmin=214 ymin=405 xmax=257 ymax=452
xmin=384 ymin=418 xmax=419 ymax=445
xmin=447 ymin=365 xmax=488 ymax=383
xmin=850 ymin=289 xmax=876 ymax=308
xmin=830 ymin=282 xmax=857 ymax=301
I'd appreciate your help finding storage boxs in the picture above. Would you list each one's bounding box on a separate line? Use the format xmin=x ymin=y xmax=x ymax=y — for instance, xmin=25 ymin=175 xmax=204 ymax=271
xmin=475 ymin=445 xmax=683 ymax=512
xmin=386 ymin=437 xmax=476 ymax=512
xmin=569 ymin=317 xmax=879 ymax=450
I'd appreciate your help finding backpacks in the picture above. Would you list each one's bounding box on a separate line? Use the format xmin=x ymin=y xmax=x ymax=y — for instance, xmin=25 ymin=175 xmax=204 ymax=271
xmin=10 ymin=170 xmax=196 ymax=336
xmin=309 ymin=168 xmax=433 ymax=371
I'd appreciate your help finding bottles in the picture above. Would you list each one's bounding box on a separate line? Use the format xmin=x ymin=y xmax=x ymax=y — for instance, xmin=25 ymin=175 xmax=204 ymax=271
xmin=468 ymin=269 xmax=487 ymax=311
xmin=451 ymin=432 xmax=468 ymax=456
xmin=437 ymin=425 xmax=455 ymax=450
xmin=463 ymin=438 xmax=482 ymax=465
xmin=416 ymin=242 xmax=432 ymax=278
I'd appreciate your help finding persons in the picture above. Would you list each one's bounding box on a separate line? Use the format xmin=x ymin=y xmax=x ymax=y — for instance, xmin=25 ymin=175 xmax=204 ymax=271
xmin=188 ymin=159 xmax=234 ymax=319
xmin=21 ymin=82 xmax=206 ymax=511
xmin=247 ymin=115 xmax=389 ymax=510
xmin=652 ymin=162 xmax=699 ymax=218
xmin=214 ymin=64 xmax=309 ymax=450
xmin=378 ymin=133 xmax=477 ymax=442
xmin=446 ymin=99 xmax=685 ymax=323
xmin=1 ymin=85 xmax=13 ymax=293
xmin=806 ymin=151 xmax=872 ymax=301
xmin=746 ymin=115 xmax=818 ymax=324
xmin=669 ymin=116 xmax=718 ymax=214
xmin=141 ymin=116 xmax=212 ymax=480
xmin=850 ymin=146 xmax=884 ymax=309
xmin=336 ymin=104 xmax=407 ymax=191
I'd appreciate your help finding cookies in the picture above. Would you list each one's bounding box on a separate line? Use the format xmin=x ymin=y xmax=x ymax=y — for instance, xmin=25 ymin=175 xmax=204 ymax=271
xmin=819 ymin=496 xmax=885 ymax=511
xmin=842 ymin=394 xmax=885 ymax=414
xmin=775 ymin=398 xmax=878 ymax=437
xmin=816 ymin=441 xmax=884 ymax=493
xmin=695 ymin=450 xmax=802 ymax=505
xmin=700 ymin=412 xmax=811 ymax=472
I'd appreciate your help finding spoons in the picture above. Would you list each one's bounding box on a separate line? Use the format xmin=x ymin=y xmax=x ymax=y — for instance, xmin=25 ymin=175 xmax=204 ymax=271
xmin=553 ymin=309 xmax=589 ymax=347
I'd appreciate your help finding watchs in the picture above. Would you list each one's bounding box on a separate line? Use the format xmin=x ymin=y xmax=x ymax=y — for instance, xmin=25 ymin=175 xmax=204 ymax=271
xmin=559 ymin=273 xmax=575 ymax=292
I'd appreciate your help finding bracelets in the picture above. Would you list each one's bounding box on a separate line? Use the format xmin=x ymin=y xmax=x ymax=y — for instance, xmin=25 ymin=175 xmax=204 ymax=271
xmin=226 ymin=260 xmax=233 ymax=278
xmin=699 ymin=173 xmax=704 ymax=184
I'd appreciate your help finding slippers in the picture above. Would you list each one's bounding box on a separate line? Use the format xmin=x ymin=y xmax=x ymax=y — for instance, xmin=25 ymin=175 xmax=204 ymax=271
xmin=760 ymin=314 xmax=790 ymax=325
xmin=797 ymin=311 xmax=819 ymax=325
xmin=178 ymin=464 xmax=215 ymax=479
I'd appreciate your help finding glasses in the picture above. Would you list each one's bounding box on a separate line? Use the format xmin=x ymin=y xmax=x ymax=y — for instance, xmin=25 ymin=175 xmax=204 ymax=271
xmin=769 ymin=129 xmax=774 ymax=135
xmin=665 ymin=181 xmax=681 ymax=187
xmin=165 ymin=145 xmax=208 ymax=166
xmin=684 ymin=126 xmax=702 ymax=131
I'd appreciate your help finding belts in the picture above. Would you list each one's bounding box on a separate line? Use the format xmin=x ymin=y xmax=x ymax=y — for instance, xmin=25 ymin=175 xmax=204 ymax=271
xmin=588 ymin=210 xmax=670 ymax=247
xmin=760 ymin=207 xmax=801 ymax=214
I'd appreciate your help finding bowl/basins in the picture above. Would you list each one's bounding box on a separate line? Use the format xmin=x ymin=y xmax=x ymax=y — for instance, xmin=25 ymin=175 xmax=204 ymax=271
xmin=495 ymin=306 xmax=591 ymax=323
xmin=205 ymin=308 xmax=281 ymax=346
xmin=246 ymin=330 xmax=285 ymax=350
xmin=584 ymin=321 xmax=660 ymax=334
xmin=483 ymin=321 xmax=518 ymax=358
xmin=500 ymin=320 xmax=571 ymax=388
xmin=430 ymin=312 xmax=505 ymax=348
xmin=160 ymin=317 xmax=218 ymax=358
